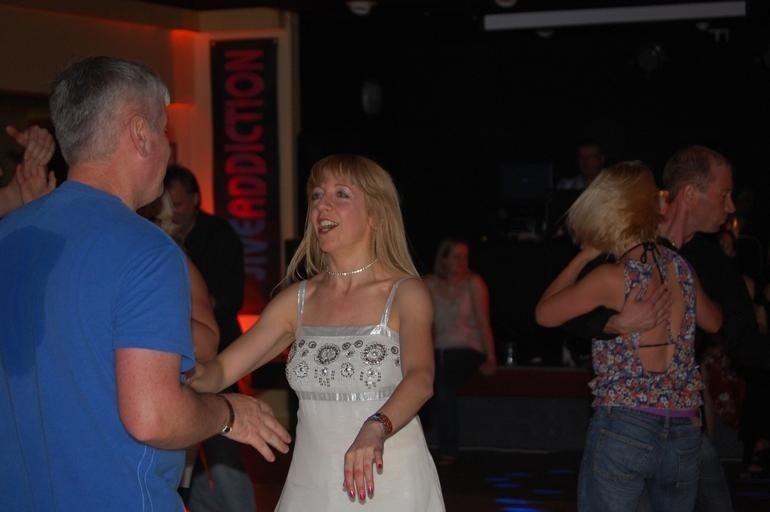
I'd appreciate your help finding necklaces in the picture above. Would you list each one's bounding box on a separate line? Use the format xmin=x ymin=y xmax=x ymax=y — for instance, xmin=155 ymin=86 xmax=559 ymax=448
xmin=320 ymin=259 xmax=380 ymax=277
xmin=437 ymin=278 xmax=467 ymax=294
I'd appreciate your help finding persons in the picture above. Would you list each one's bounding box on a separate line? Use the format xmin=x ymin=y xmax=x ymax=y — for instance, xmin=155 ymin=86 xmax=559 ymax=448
xmin=0 ymin=56 xmax=293 ymax=511
xmin=534 ymin=138 xmax=769 ymax=512
xmin=184 ymin=153 xmax=444 ymax=512
xmin=419 ymin=236 xmax=497 ymax=466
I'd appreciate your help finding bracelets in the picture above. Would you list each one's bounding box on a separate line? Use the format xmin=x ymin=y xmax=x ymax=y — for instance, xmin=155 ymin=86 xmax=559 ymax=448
xmin=362 ymin=412 xmax=393 ymax=439
